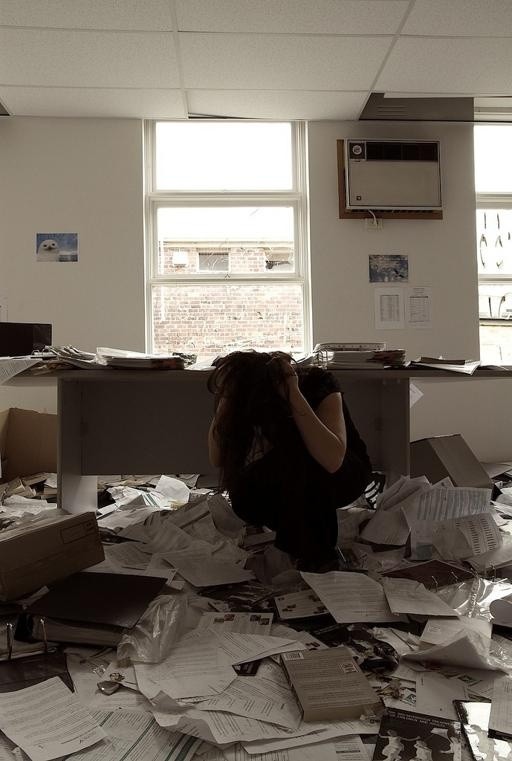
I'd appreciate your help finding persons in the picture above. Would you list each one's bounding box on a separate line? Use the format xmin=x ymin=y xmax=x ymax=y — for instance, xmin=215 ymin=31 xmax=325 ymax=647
xmin=378 ymin=729 xmax=422 ymax=761
xmin=496 ymin=739 xmax=512 ymax=760
xmin=439 ymin=726 xmax=461 ymax=761
xmin=408 ymin=739 xmax=433 ymax=761
xmin=207 ymin=350 xmax=372 ymax=574
xmin=465 ymin=723 xmax=500 ymax=760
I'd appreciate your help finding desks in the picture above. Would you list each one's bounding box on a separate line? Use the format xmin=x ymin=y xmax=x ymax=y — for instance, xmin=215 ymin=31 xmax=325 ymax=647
xmin=5 ymin=364 xmax=509 ymax=517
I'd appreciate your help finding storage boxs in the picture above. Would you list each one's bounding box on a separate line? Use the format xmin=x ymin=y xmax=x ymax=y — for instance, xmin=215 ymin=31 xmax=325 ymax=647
xmin=0 ymin=504 xmax=107 ymax=606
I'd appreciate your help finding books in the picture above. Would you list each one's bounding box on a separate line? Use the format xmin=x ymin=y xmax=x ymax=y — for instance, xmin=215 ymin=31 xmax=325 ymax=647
xmin=452 ymin=698 xmax=512 ymax=761
xmin=0 ymin=344 xmax=215 ymax=374
xmin=485 ymin=675 xmax=512 ymax=739
xmin=381 ymin=560 xmax=474 ymax=592
xmin=44 ymin=496 xmax=57 ymax=503
xmin=368 ymin=706 xmax=464 ymax=761
xmin=279 ymin=648 xmax=382 ymax=724
xmin=290 ymin=342 xmax=507 ymax=374
xmin=30 ymin=494 xmax=57 ymax=500
xmin=28 ymin=479 xmax=57 ymax=494
